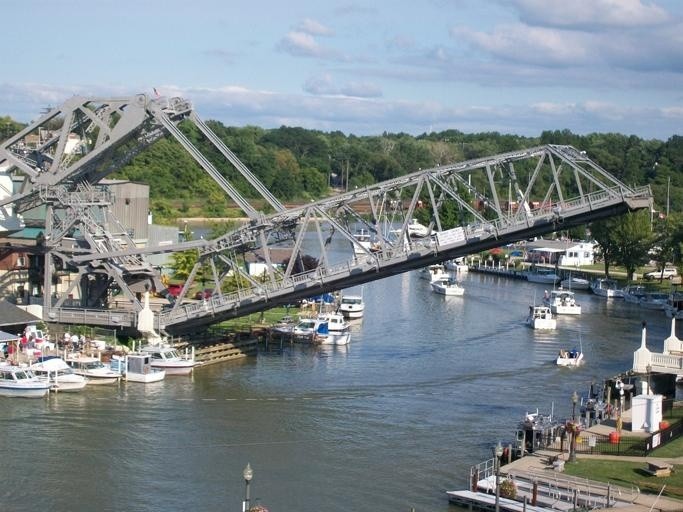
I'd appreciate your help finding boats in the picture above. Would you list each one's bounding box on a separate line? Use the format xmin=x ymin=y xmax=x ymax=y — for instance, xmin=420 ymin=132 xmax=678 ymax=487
xmin=389 ymin=218 xmax=438 ymax=239
xmin=0 ymin=345 xmax=197 ymax=400
xmin=350 ymin=227 xmax=374 ymax=260
xmin=273 ymin=294 xmax=365 ymax=346
xmin=418 ymin=257 xmax=469 ymax=297
xmin=556 ymin=348 xmax=584 ymax=366
xmin=526 ymin=267 xmax=683 ymax=330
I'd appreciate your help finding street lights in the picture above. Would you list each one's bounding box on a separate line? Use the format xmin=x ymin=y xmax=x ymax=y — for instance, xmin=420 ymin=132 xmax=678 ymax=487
xmin=494 ymin=439 xmax=504 ymax=512
xmin=242 ymin=461 xmax=253 ymax=512
xmin=571 ymin=391 xmax=579 ymax=421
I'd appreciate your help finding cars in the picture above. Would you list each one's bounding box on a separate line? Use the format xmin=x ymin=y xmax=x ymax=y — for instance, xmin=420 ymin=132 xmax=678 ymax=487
xmin=194 ymin=289 xmax=213 ymax=300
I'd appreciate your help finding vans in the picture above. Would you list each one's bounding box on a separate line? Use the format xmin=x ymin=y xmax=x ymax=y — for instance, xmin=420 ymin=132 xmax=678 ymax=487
xmin=166 ymin=283 xmax=185 ymax=296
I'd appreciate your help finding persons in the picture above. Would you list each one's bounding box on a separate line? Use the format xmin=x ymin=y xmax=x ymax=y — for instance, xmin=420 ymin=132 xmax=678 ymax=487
xmin=3 ymin=330 xmax=86 ymax=359
xmin=615 ymin=415 xmax=622 ymax=433
